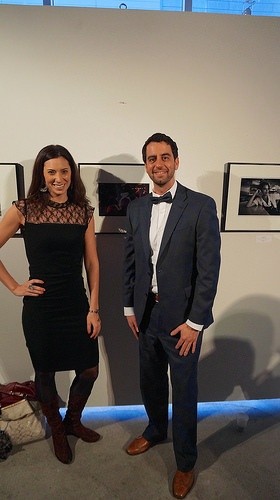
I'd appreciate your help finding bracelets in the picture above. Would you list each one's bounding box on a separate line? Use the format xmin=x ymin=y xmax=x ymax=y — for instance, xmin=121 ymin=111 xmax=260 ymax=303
xmin=89 ymin=308 xmax=100 ymax=313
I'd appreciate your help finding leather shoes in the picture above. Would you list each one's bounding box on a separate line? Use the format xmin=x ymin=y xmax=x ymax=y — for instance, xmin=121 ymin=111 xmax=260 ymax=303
xmin=125 ymin=435 xmax=152 ymax=456
xmin=172 ymin=471 xmax=194 ymax=498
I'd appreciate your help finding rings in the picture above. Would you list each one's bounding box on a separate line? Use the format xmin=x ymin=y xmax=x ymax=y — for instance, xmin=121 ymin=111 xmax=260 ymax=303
xmin=28 ymin=284 xmax=34 ymax=289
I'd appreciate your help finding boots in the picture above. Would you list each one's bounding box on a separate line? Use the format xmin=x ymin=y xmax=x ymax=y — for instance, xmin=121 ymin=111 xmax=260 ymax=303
xmin=40 ymin=399 xmax=73 ymax=465
xmin=63 ymin=389 xmax=100 ymax=443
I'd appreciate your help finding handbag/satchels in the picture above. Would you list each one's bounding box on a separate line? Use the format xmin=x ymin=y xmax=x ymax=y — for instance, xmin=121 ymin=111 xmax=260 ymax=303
xmin=0 ymin=381 xmax=40 ymax=407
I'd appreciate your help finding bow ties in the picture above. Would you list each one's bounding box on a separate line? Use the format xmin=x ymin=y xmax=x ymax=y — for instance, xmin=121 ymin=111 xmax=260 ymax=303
xmin=149 ymin=191 xmax=172 ymax=204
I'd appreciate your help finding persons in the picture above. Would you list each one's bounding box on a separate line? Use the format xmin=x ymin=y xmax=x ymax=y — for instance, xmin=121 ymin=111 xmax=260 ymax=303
xmin=246 ymin=181 xmax=277 ymax=215
xmin=0 ymin=145 xmax=102 ymax=464
xmin=122 ymin=133 xmax=221 ymax=497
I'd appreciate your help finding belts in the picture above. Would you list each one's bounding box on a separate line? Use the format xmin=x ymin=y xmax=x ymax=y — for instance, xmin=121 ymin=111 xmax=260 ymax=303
xmin=151 ymin=292 xmax=159 ymax=301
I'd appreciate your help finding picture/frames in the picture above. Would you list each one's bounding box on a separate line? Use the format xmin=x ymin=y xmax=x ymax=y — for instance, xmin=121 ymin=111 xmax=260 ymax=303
xmin=0 ymin=163 xmax=26 ymax=239
xmin=76 ymin=162 xmax=154 ymax=234
xmin=220 ymin=162 xmax=279 ymax=233
